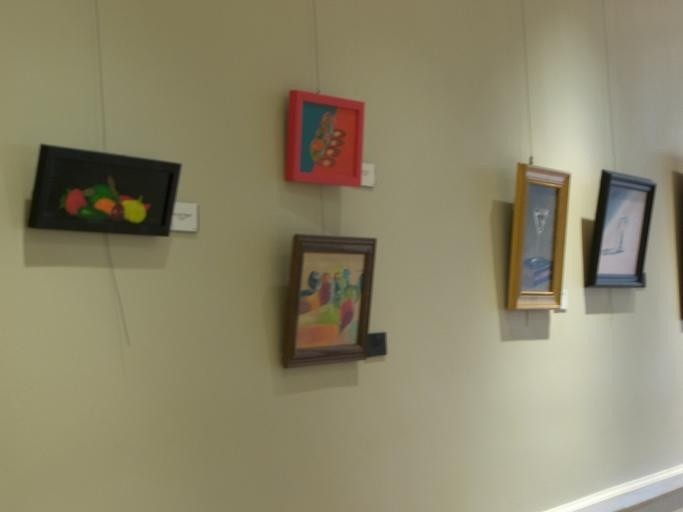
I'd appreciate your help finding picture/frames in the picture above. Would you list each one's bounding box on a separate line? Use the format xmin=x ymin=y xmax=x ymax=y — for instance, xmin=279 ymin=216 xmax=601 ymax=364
xmin=27 ymin=143 xmax=181 ymax=237
xmin=280 ymin=234 xmax=377 ymax=369
xmin=285 ymin=90 xmax=365 ymax=188
xmin=506 ymin=161 xmax=571 ymax=311
xmin=584 ymin=169 xmax=656 ymax=288
